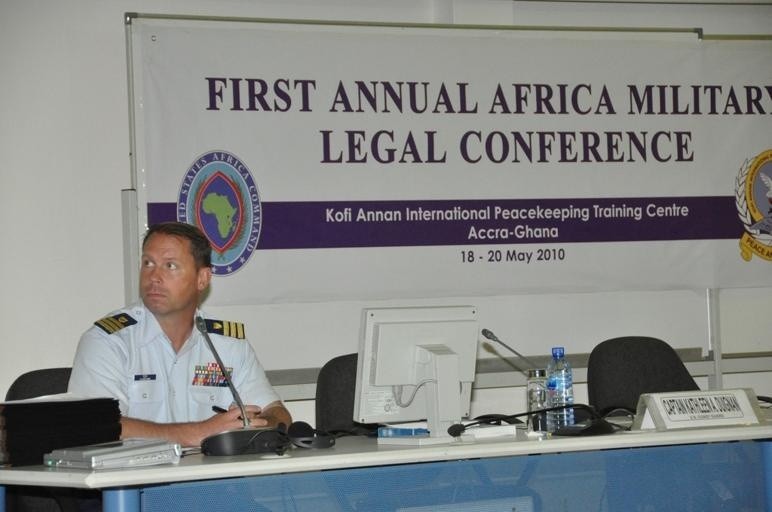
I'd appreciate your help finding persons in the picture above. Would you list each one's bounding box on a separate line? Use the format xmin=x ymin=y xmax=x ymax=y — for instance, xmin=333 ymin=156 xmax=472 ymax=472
xmin=66 ymin=221 xmax=292 ymax=448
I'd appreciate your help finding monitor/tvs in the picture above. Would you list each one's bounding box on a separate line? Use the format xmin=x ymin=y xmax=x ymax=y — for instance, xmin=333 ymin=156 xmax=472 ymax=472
xmin=352 ymin=305 xmax=516 ymax=446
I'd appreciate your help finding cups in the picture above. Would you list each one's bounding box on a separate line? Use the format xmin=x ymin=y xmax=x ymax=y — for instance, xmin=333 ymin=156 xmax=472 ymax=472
xmin=526 ymin=370 xmax=547 ymax=432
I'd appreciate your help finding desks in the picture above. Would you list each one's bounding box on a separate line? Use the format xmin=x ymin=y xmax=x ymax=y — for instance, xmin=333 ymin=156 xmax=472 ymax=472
xmin=0 ymin=406 xmax=772 ymax=512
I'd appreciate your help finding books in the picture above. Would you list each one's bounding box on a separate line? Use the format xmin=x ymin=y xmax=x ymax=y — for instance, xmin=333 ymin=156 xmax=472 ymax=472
xmin=377 ymin=421 xmax=517 ymax=439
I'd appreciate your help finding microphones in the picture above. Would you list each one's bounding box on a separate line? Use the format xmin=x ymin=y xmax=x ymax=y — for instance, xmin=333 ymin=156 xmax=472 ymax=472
xmin=447 ymin=403 xmax=614 ymax=437
xmin=481 ymin=328 xmax=540 ymax=369
xmin=195 ymin=316 xmax=290 ymax=456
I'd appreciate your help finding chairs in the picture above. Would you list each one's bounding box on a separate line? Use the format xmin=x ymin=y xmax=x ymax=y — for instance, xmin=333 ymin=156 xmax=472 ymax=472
xmin=586 ymin=336 xmax=702 ymax=415
xmin=4 ymin=367 xmax=73 ymax=401
xmin=315 ymin=352 xmax=379 ymax=437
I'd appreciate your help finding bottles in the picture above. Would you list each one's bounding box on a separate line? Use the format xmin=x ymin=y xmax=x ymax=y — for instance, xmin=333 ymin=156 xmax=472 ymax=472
xmin=545 ymin=348 xmax=573 ymax=434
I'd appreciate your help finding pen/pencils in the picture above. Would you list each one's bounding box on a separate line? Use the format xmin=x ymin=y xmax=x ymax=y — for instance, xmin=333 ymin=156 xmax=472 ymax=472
xmin=211 ymin=406 xmax=252 ymax=422
